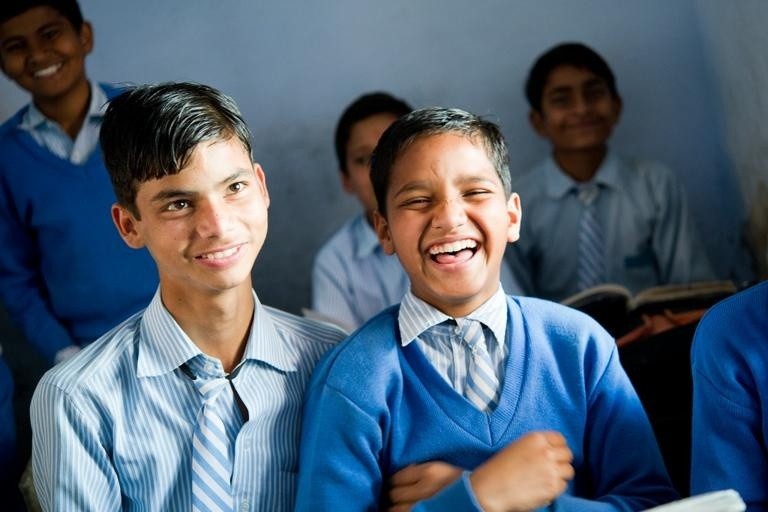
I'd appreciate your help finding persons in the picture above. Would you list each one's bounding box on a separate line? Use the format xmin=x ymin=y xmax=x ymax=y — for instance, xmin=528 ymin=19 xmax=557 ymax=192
xmin=308 ymin=90 xmax=527 ymax=337
xmin=507 ymin=39 xmax=722 ymax=319
xmin=290 ymin=105 xmax=684 ymax=510
xmin=0 ymin=1 xmax=162 ymax=368
xmin=29 ymin=76 xmax=353 ymax=511
xmin=683 ymin=274 xmax=768 ymax=512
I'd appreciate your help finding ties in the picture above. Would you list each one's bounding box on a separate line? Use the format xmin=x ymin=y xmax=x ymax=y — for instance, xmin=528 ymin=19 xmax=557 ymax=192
xmin=576 ymin=186 xmax=606 ymax=291
xmin=460 ymin=321 xmax=499 ymax=414
xmin=191 ymin=375 xmax=233 ymax=512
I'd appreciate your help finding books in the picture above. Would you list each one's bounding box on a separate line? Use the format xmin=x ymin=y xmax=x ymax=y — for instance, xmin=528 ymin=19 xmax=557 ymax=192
xmin=558 ymin=279 xmax=740 ymax=311
